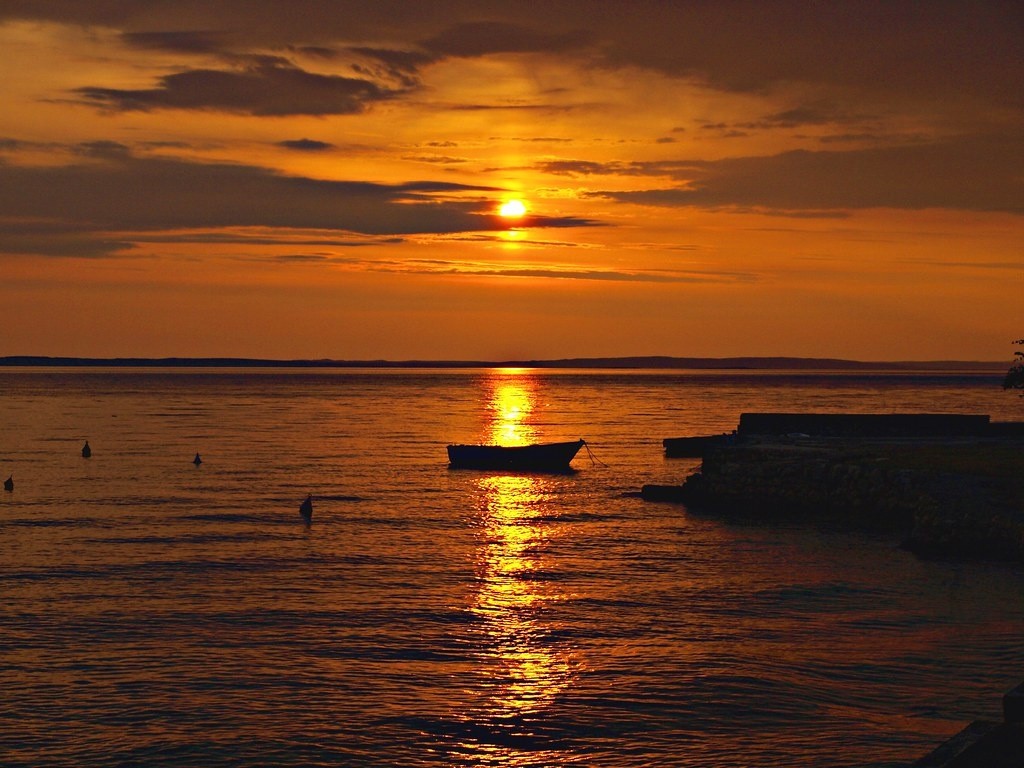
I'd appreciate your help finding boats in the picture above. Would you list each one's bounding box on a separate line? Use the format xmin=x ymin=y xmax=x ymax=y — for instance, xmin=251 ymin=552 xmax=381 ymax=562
xmin=446 ymin=438 xmax=587 ymax=470
